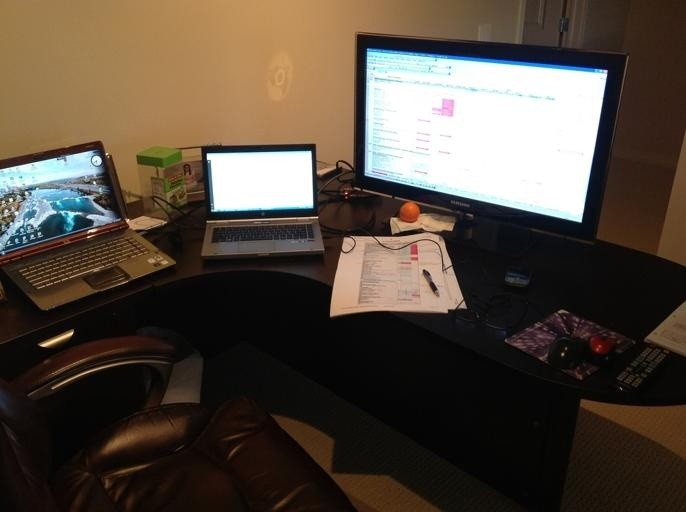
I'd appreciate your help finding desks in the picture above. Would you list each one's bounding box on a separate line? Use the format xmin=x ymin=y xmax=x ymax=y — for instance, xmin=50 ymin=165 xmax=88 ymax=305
xmin=0 ymin=171 xmax=686 ymax=511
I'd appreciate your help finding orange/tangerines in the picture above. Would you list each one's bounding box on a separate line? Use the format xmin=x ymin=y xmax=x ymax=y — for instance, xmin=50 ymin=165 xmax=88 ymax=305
xmin=399 ymin=202 xmax=420 ymax=223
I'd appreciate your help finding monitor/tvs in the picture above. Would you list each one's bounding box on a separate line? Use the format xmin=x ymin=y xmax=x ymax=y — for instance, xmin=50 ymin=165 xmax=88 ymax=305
xmin=352 ymin=30 xmax=631 ymax=269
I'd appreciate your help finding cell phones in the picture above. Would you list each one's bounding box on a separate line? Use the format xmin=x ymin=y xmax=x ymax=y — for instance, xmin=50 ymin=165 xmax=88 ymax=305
xmin=504 ymin=267 xmax=536 ymax=289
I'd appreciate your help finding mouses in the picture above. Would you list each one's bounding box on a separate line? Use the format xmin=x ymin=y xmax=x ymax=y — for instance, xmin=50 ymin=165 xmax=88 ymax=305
xmin=548 ymin=331 xmax=586 ymax=372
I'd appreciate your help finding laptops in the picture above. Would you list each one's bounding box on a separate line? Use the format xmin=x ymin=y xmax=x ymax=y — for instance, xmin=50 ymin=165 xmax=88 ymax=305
xmin=201 ymin=143 xmax=325 ymax=259
xmin=0 ymin=139 xmax=176 ymax=314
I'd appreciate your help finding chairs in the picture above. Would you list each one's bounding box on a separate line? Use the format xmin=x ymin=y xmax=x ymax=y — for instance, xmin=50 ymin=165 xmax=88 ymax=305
xmin=0 ymin=333 xmax=356 ymax=511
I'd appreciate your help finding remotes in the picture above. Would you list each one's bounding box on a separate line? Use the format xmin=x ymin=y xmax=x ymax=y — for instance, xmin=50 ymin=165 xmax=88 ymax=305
xmin=614 ymin=341 xmax=672 ymax=394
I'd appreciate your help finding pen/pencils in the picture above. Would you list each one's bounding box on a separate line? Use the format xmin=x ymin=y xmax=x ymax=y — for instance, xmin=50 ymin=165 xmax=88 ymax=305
xmin=423 ymin=269 xmax=440 ymax=297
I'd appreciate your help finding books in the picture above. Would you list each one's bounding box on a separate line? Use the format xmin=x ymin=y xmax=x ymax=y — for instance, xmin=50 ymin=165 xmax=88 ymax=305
xmin=431 ymin=248 xmax=469 ymax=314
xmin=326 ymin=231 xmax=449 ymax=318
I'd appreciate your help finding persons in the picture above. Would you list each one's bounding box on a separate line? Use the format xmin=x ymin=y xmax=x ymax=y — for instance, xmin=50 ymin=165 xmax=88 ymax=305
xmin=183 ymin=163 xmax=197 ymax=190
